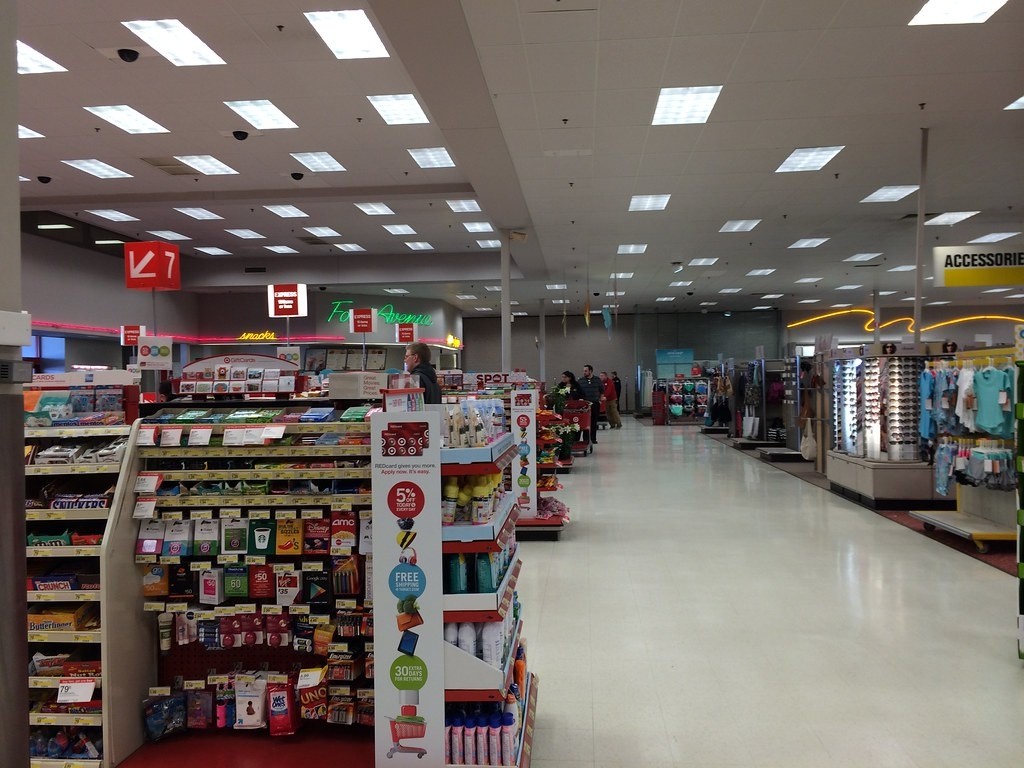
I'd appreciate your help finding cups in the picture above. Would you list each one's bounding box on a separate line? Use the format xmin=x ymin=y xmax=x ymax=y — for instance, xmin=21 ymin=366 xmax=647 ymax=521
xmin=253 ymin=527 xmax=271 ymax=549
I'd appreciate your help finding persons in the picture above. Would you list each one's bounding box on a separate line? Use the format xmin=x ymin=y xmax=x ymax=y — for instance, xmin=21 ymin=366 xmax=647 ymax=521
xmin=599 ymin=372 xmax=622 ymax=429
xmin=883 ymin=342 xmax=897 ymax=354
xmin=577 ymin=364 xmax=604 ymax=444
xmin=403 ymin=341 xmax=442 ymax=404
xmin=942 ymin=341 xmax=956 ymax=353
xmin=555 ymin=371 xmax=584 ymax=401
xmin=610 ymin=371 xmax=621 ymax=415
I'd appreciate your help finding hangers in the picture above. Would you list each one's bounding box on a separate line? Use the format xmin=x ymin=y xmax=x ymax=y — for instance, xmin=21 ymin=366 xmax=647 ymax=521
xmin=917 ymin=356 xmax=1019 ymax=457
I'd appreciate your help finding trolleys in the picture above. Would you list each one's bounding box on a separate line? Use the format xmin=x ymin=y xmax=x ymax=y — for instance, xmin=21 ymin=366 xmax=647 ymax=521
xmin=596 ymin=397 xmax=608 ymax=430
xmin=562 ymin=399 xmax=593 ymax=456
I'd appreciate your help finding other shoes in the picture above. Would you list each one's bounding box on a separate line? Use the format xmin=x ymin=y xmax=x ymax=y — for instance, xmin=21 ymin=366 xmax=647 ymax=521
xmin=610 ymin=426 xmax=615 ymax=429
xmin=616 ymin=424 xmax=622 ymax=429
xmin=592 ymin=441 xmax=598 ymax=444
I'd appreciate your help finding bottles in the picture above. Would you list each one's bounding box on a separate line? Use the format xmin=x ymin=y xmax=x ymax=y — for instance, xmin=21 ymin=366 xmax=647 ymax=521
xmin=444 ymin=638 xmax=527 ymax=766
xmin=448 ymin=526 xmax=515 ymax=594
xmin=441 ymin=470 xmax=506 ymax=527
xmin=442 ymin=595 xmax=519 ymax=671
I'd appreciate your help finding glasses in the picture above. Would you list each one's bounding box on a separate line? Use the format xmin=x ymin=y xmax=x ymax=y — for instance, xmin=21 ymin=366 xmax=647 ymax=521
xmin=830 ymin=358 xmax=925 ymax=446
xmin=404 ymin=353 xmax=416 ymax=359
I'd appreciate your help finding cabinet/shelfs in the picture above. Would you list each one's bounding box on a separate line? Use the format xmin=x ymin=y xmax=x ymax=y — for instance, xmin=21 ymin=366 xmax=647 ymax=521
xmin=22 ymin=353 xmax=573 ymax=768
xmin=906 ymin=345 xmax=1024 ymax=554
xmin=652 ymin=322 xmax=956 ymax=510
